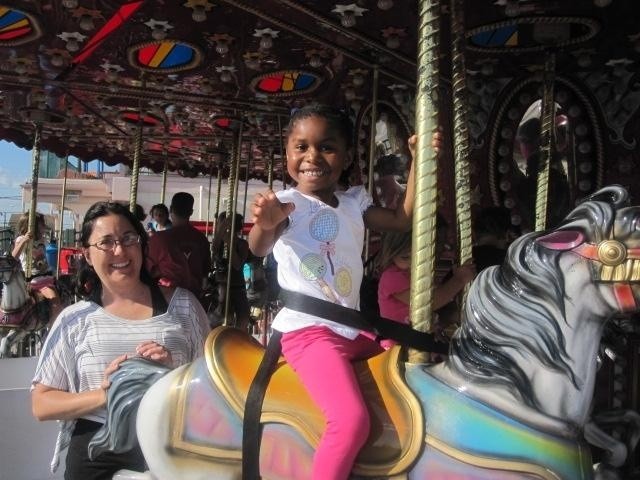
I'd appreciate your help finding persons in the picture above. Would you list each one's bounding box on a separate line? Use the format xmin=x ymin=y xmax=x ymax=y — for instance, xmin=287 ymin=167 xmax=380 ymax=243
xmin=249 ymin=104 xmax=442 ymax=480
xmin=127 ymin=192 xmax=280 ymax=334
xmin=377 ymin=230 xmax=478 ymax=324
xmin=12 ymin=211 xmax=60 ymax=343
xmin=46 ymin=236 xmax=58 ymax=271
xmin=29 ymin=201 xmax=212 ymax=480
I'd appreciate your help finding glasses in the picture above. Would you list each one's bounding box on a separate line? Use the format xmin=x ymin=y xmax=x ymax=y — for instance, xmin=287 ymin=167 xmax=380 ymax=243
xmin=84 ymin=232 xmax=142 ymax=252
